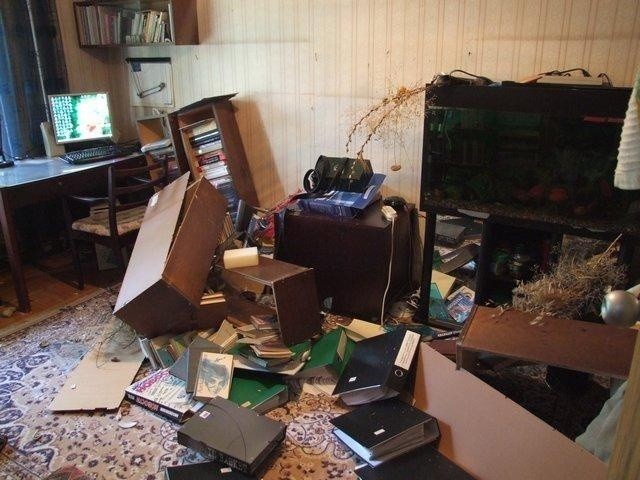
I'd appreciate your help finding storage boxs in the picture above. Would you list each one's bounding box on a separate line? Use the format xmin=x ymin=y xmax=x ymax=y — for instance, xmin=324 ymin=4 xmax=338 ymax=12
xmin=410 ymin=307 xmax=638 ymax=478
xmin=114 ymin=170 xmax=228 ymax=338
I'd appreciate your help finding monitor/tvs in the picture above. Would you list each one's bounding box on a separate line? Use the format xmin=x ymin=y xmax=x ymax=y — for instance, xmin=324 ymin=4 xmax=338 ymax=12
xmin=47 ymin=91 xmax=114 ymax=152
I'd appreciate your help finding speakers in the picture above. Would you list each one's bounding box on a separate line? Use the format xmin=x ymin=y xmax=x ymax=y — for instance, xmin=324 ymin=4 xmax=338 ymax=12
xmin=109 ymin=126 xmax=122 ymax=144
xmin=40 ymin=121 xmax=66 ymax=157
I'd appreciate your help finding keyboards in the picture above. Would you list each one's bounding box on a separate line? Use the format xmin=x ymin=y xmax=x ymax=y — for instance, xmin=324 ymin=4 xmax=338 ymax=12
xmin=64 ymin=143 xmax=133 ymax=165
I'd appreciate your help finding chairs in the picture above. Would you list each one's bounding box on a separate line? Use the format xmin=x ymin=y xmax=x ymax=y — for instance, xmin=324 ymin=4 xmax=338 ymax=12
xmin=70 ymin=153 xmax=169 ymax=291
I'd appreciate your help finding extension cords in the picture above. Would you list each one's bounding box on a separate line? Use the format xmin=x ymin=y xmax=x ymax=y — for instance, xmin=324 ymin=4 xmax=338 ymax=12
xmin=536 ymin=75 xmax=603 ymax=86
xmin=381 ymin=205 xmax=399 ymax=222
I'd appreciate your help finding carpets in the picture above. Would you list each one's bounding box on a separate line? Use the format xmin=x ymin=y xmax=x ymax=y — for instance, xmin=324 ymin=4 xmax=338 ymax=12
xmin=2 ymin=282 xmax=417 ymax=478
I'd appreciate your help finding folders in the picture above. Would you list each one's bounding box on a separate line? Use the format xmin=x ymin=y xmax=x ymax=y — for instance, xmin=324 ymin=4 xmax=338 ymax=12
xmin=298 ymin=327 xmax=356 ymax=377
xmin=228 ymin=371 xmax=290 ymax=416
xmin=332 ymin=330 xmax=422 ymax=407
xmin=329 ymin=398 xmax=441 ymax=470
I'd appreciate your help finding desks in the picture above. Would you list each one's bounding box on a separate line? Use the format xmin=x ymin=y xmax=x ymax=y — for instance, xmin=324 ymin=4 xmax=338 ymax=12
xmin=0 ymin=151 xmax=146 ymax=314
xmin=456 ymin=303 xmax=639 ymax=391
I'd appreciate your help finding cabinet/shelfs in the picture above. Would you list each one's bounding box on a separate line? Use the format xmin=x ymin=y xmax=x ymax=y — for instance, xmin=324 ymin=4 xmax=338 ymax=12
xmin=136 ymin=111 xmax=186 ymax=192
xmin=176 ymin=92 xmax=261 ymax=232
xmin=275 ymin=200 xmax=417 ymax=325
xmin=419 ymin=207 xmax=637 ymax=334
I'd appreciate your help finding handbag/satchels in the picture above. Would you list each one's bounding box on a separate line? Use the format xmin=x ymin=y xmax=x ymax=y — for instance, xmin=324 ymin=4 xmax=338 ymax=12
xmin=311 ymin=155 xmax=374 ymax=194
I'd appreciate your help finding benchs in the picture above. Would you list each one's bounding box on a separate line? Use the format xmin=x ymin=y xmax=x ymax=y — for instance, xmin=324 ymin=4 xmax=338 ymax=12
xmin=213 ymin=253 xmax=321 ymax=345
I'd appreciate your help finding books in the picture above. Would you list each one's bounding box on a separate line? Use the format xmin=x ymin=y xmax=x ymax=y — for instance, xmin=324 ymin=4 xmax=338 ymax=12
xmin=72 ymin=3 xmax=172 ymax=45
xmin=125 ymin=190 xmax=477 ymax=479
xmin=178 ymin=118 xmax=235 ymax=187
xmin=140 ymin=138 xmax=178 ymax=187
